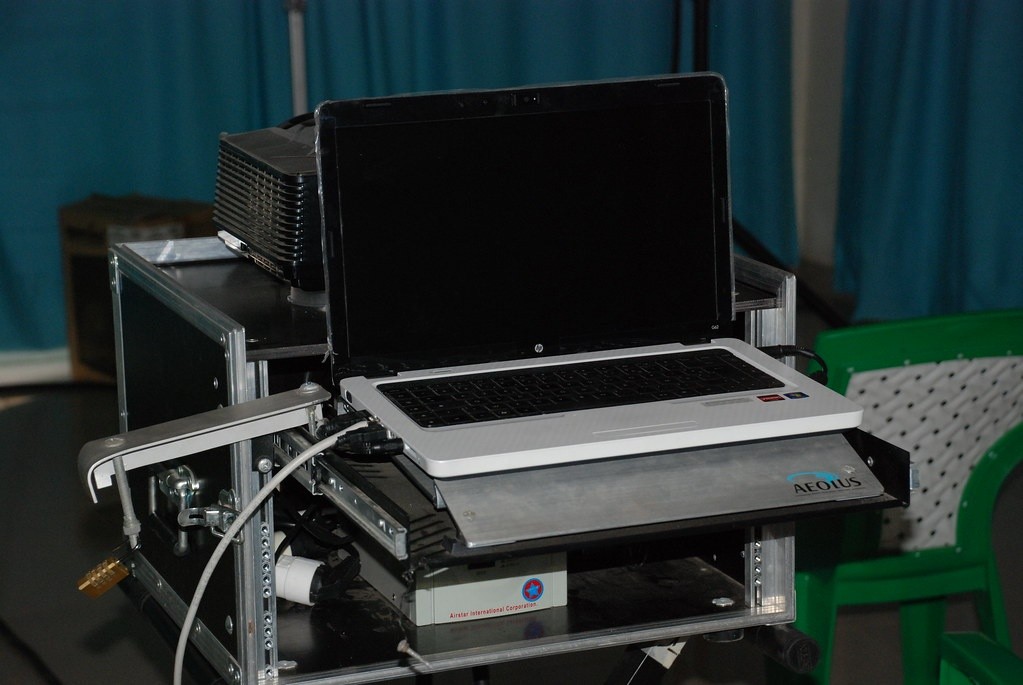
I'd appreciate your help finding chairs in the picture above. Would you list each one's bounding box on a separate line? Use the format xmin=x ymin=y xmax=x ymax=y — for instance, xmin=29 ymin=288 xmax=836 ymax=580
xmin=792 ymin=315 xmax=1023 ymax=685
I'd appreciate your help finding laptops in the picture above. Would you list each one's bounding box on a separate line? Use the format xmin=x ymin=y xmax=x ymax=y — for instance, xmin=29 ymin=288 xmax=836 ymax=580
xmin=313 ymin=70 xmax=864 ymax=481
